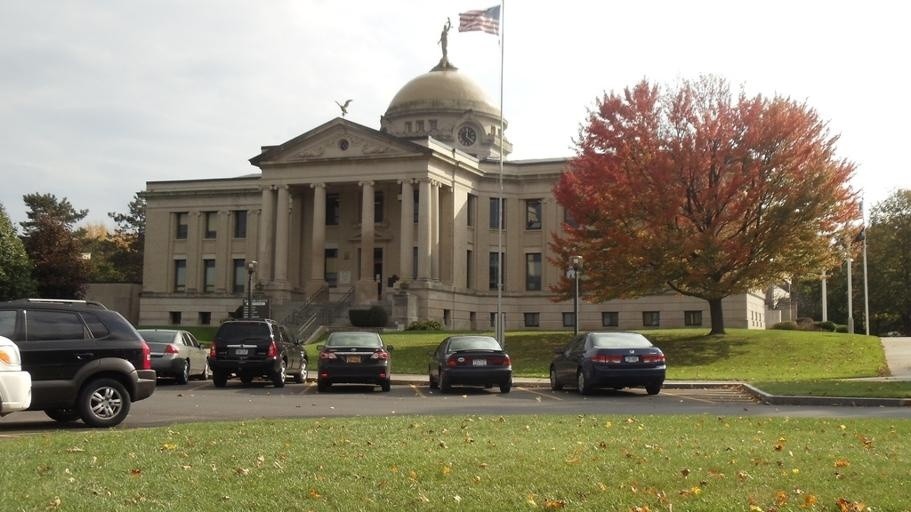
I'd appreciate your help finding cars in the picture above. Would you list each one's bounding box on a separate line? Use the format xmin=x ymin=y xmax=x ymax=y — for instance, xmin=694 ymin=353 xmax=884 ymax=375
xmin=134 ymin=327 xmax=212 ymax=385
xmin=315 ymin=329 xmax=400 ymax=393
xmin=0 ymin=338 xmax=40 ymax=419
xmin=427 ymin=335 xmax=512 ymax=394
xmin=550 ymin=329 xmax=668 ymax=395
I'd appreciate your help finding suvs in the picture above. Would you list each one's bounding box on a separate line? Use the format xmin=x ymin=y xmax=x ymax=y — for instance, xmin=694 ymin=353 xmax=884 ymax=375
xmin=205 ymin=315 xmax=309 ymax=387
xmin=0 ymin=295 xmax=157 ymax=425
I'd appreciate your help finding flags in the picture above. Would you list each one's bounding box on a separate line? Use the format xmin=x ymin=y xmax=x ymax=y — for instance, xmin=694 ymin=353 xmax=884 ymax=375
xmin=456 ymin=5 xmax=502 ymax=38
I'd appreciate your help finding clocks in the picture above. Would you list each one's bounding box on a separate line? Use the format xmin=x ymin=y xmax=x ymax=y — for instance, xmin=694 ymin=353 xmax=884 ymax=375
xmin=456 ymin=124 xmax=478 ymax=149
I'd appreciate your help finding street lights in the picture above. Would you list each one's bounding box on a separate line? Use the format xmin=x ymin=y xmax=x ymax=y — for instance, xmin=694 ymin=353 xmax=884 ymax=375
xmin=245 ymin=259 xmax=256 ymax=319
xmin=568 ymin=254 xmax=586 ymax=338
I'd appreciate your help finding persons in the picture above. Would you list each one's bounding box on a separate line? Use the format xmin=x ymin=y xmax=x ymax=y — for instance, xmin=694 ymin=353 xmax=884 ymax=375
xmin=436 ymin=16 xmax=452 ymax=65
